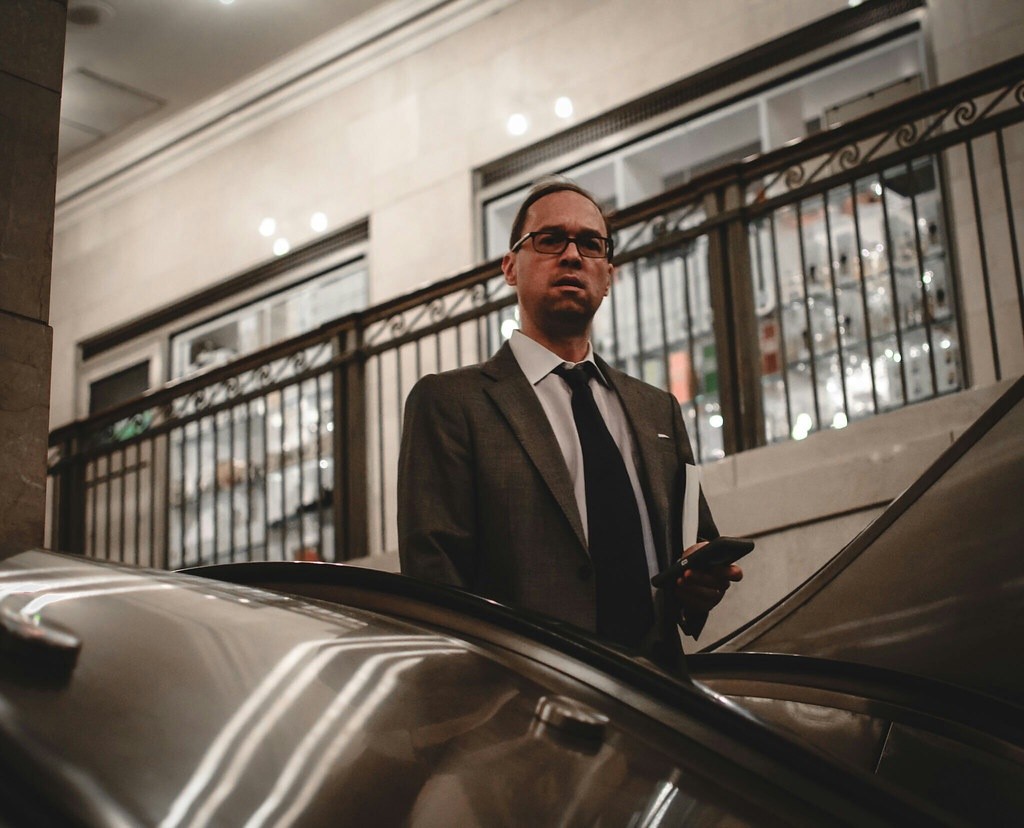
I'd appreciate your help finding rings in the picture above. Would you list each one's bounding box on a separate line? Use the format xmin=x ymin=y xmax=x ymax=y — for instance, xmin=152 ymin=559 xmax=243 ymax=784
xmin=713 ymin=590 xmax=722 ymax=601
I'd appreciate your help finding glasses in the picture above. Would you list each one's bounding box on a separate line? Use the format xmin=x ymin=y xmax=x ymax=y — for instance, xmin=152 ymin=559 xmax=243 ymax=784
xmin=511 ymin=232 xmax=614 ymax=263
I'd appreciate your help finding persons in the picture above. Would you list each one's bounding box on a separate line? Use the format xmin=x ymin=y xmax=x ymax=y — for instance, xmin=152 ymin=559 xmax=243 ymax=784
xmin=399 ymin=184 xmax=743 ymax=665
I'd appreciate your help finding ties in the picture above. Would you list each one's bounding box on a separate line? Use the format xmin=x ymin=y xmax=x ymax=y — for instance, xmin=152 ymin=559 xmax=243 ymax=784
xmin=551 ymin=362 xmax=655 ymax=650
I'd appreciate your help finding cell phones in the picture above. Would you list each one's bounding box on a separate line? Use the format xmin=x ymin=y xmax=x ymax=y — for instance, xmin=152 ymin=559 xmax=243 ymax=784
xmin=653 ymin=536 xmax=756 ymax=589
